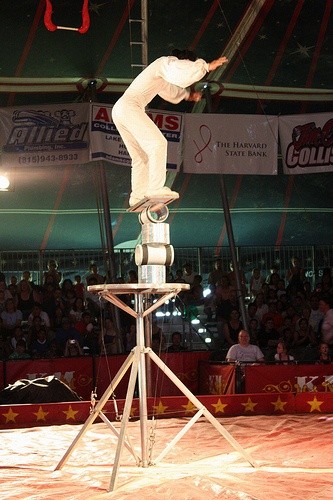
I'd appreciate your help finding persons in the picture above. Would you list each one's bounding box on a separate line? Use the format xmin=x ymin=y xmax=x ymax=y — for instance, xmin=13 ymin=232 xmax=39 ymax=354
xmin=274 ymin=340 xmax=295 ymax=365
xmin=112 ymin=44 xmax=228 ymax=208
xmin=226 ymin=330 xmax=264 ymax=366
xmin=0 ymin=260 xmax=332 ymax=361
xmin=314 ymin=344 xmax=331 ymax=363
